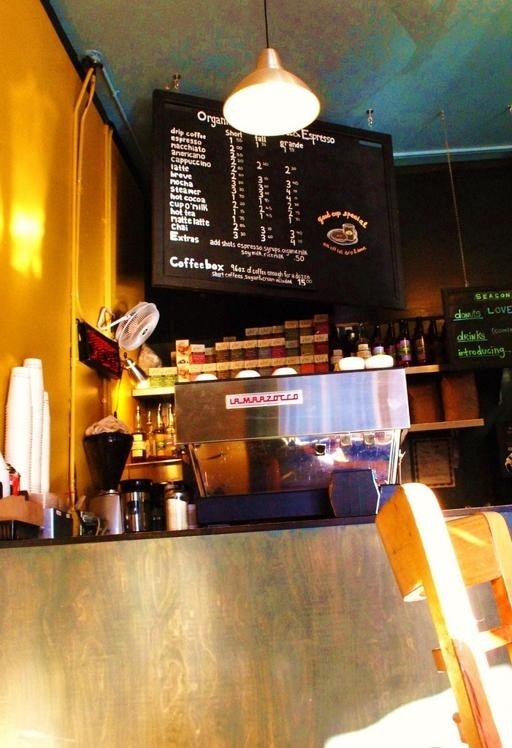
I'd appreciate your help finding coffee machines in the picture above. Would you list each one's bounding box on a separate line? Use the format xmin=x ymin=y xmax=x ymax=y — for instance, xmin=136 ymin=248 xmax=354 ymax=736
xmin=82 ymin=431 xmax=133 ymax=536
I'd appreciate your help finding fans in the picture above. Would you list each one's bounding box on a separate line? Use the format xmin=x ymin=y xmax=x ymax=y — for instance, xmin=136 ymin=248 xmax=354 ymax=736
xmin=96 ymin=301 xmax=160 ymax=352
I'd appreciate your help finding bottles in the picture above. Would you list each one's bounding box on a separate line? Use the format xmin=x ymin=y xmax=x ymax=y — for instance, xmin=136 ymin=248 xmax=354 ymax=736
xmin=427 ymin=318 xmax=443 ymax=365
xmin=129 ymin=405 xmax=147 ymax=463
xmin=355 ymin=325 xmax=372 ymax=360
xmin=371 ymin=320 xmax=385 ymax=356
xmin=331 ymin=326 xmax=351 ymax=373
xmin=381 ymin=320 xmax=396 ymax=369
xmin=144 ymin=409 xmax=157 ymax=461
xmin=412 ymin=316 xmax=429 ymax=366
xmin=153 ymin=403 xmax=167 ymax=460
xmin=164 ymin=403 xmax=179 ymax=459
xmin=395 ymin=318 xmax=413 ymax=368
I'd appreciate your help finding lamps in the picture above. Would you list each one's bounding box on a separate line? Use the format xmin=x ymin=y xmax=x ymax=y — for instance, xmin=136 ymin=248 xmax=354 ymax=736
xmin=222 ymin=0 xmax=321 ymax=136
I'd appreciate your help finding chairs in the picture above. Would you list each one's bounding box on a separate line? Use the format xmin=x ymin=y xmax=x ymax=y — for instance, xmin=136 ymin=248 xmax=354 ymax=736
xmin=375 ymin=482 xmax=512 ymax=748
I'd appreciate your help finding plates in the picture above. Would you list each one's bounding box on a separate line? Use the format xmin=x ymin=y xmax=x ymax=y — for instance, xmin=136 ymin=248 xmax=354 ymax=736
xmin=327 ymin=229 xmax=358 ymax=246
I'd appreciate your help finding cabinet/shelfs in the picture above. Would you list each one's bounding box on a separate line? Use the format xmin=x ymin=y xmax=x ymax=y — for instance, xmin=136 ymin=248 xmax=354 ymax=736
xmin=125 ymin=364 xmax=485 ymax=483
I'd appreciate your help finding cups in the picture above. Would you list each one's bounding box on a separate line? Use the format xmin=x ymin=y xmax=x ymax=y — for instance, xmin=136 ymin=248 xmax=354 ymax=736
xmin=186 ymin=502 xmax=198 ymax=529
xmin=118 ymin=478 xmax=155 ymax=533
xmin=164 ymin=483 xmax=188 ymax=532
xmin=342 ymin=222 xmax=356 ymax=241
xmin=0 ymin=356 xmax=51 ymax=508
